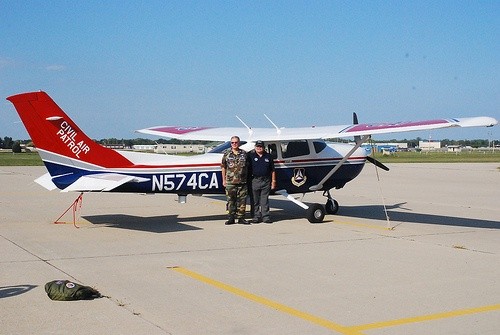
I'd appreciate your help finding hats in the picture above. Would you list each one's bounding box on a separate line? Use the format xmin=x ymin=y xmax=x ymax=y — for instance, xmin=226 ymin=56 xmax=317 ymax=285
xmin=256 ymin=141 xmax=264 ymax=146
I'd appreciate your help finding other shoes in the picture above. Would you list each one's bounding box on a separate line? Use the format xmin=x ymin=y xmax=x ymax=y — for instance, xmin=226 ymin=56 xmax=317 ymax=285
xmin=262 ymin=215 xmax=273 ymax=223
xmin=225 ymin=218 xmax=235 ymax=224
xmin=252 ymin=216 xmax=261 ymax=223
xmin=238 ymin=218 xmax=250 ymax=224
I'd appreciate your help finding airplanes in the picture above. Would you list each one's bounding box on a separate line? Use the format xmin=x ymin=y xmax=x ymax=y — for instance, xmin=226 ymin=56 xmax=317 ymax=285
xmin=5 ymin=89 xmax=499 ymax=225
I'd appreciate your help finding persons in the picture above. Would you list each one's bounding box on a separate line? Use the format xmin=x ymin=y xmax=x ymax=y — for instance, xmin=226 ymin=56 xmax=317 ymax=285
xmin=247 ymin=139 xmax=277 ymax=224
xmin=220 ymin=135 xmax=251 ymax=225
